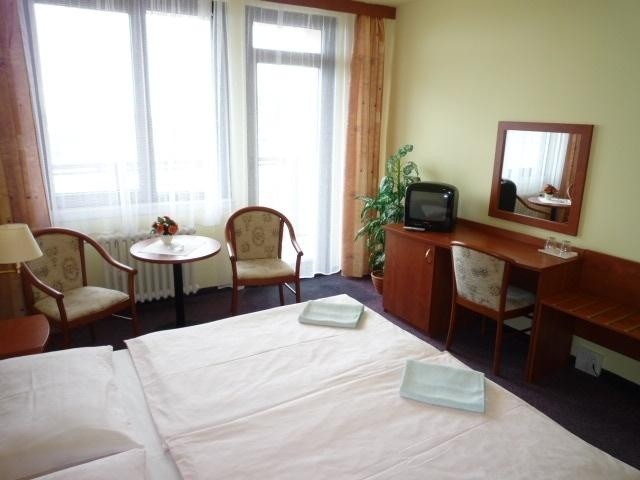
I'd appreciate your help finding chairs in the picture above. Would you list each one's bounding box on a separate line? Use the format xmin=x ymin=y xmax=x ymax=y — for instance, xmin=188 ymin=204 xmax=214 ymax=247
xmin=19 ymin=228 xmax=143 ymax=352
xmin=444 ymin=239 xmax=537 ymax=376
xmin=224 ymin=206 xmax=304 ymax=316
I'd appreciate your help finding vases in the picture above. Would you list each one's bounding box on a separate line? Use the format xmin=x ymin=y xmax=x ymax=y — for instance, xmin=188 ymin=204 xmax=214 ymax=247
xmin=161 ymin=235 xmax=174 ymax=244
xmin=544 ymin=193 xmax=553 ymax=200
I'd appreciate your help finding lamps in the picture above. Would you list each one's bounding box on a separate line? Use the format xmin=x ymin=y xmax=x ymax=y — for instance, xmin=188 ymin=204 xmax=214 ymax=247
xmin=0 ymin=222 xmax=44 ymax=275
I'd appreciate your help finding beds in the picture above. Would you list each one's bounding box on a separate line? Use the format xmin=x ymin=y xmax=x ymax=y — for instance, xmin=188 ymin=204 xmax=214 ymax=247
xmin=0 ymin=293 xmax=640 ymax=480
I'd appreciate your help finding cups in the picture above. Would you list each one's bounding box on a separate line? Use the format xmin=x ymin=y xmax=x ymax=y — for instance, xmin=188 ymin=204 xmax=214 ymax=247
xmin=544 ymin=237 xmax=557 ymax=252
xmin=560 ymin=240 xmax=571 ymax=257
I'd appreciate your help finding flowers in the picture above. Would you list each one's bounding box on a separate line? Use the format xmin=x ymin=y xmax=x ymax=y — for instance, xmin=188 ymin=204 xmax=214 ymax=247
xmin=150 ymin=216 xmax=179 ymax=236
xmin=545 ymin=184 xmax=559 ymax=195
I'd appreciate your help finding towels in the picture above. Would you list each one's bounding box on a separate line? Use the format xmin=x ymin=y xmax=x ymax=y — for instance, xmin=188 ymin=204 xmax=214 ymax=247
xmin=397 ymin=358 xmax=486 ymax=414
xmin=298 ymin=300 xmax=365 ymax=328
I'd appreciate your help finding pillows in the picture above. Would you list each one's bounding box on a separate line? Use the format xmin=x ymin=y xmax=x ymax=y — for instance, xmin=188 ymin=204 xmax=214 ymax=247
xmin=0 ymin=344 xmax=151 ymax=480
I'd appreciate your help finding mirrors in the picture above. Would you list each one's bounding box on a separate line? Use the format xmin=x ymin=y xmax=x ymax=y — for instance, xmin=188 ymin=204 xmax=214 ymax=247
xmin=488 ymin=120 xmax=594 ymax=237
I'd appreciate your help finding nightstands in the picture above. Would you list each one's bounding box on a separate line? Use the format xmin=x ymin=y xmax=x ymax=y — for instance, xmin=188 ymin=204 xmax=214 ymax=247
xmin=0 ymin=314 xmax=51 ymax=359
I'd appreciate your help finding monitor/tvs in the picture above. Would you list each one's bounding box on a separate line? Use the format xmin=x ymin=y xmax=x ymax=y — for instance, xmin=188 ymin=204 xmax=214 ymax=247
xmin=405 ymin=181 xmax=459 ymax=232
xmin=499 ymin=179 xmax=516 ymax=211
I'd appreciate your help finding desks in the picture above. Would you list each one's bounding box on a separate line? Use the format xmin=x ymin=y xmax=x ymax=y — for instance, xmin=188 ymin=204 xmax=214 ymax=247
xmin=528 ymin=197 xmax=572 ymax=222
xmin=381 ymin=221 xmax=584 ymax=378
xmin=129 ymin=235 xmax=222 ymax=332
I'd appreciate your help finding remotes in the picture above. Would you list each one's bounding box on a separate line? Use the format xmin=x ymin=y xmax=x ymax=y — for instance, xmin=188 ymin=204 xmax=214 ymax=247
xmin=403 ymin=226 xmax=426 ymax=231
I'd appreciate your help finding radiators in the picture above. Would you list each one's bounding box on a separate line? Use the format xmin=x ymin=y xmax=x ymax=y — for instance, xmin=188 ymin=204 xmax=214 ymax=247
xmin=98 ymin=226 xmax=200 ymax=305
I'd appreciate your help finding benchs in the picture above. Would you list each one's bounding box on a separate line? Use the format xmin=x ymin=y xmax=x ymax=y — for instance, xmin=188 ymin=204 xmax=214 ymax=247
xmin=527 ymin=289 xmax=640 ymax=381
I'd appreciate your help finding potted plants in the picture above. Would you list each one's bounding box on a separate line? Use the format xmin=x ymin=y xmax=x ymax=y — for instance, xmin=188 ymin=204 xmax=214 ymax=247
xmin=353 ymin=144 xmax=421 ymax=295
